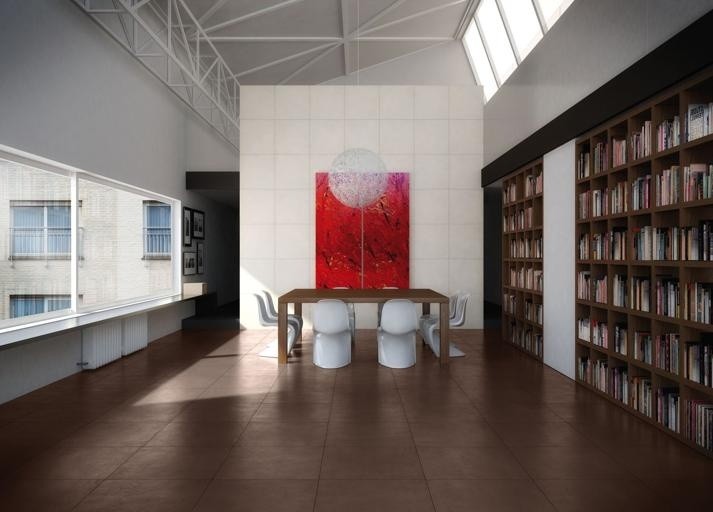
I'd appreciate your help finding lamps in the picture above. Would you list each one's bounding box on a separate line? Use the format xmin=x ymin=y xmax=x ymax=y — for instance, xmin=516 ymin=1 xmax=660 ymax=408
xmin=328 ymin=0 xmax=387 ymax=209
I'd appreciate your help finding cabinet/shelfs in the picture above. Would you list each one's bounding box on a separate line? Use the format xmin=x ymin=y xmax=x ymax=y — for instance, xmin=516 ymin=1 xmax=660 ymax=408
xmin=500 ymin=157 xmax=544 ymax=363
xmin=574 ymin=71 xmax=713 ymax=458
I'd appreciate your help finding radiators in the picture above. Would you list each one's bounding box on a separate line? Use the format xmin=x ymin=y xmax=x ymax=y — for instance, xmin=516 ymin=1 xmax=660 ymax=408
xmin=81 ymin=313 xmax=148 ymax=369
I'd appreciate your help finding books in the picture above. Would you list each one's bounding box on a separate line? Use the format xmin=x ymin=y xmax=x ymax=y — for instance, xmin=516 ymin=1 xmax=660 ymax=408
xmin=503 ymin=170 xmax=541 ymax=361
xmin=577 ymin=101 xmax=713 ymax=452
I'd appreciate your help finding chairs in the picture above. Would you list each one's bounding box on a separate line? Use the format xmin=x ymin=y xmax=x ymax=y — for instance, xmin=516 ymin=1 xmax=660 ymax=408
xmin=253 ymin=287 xmax=470 ymax=368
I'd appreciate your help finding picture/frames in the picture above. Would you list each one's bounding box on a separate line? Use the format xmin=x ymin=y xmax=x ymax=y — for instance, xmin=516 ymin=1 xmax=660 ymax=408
xmin=183 ymin=206 xmax=205 ymax=276
